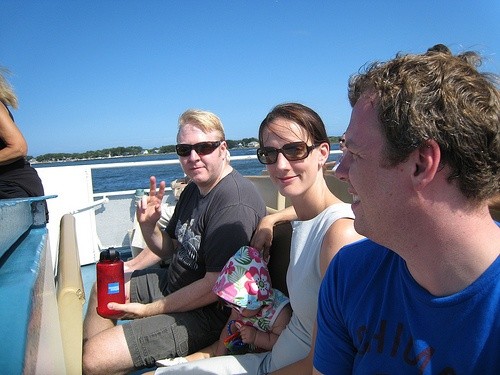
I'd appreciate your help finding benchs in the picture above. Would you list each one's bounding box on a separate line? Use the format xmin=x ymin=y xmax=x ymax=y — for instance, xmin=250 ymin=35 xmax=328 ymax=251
xmin=243 ymin=173 xmax=500 ymax=300
xmin=22 ymin=214 xmax=84 ymax=375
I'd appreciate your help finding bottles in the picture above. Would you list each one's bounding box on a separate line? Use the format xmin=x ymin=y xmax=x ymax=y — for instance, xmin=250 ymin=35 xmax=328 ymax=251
xmin=96 ymin=246 xmax=125 ymax=319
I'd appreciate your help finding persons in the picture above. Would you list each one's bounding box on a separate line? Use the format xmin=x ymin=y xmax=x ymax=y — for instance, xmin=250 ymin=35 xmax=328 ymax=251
xmin=144 ymin=102 xmax=365 ymax=375
xmin=0 ymin=76 xmax=50 ymax=200
xmin=155 ymin=248 xmax=290 ymax=367
xmin=312 ymin=43 xmax=500 ymax=375
xmin=82 ymin=110 xmax=267 ymax=375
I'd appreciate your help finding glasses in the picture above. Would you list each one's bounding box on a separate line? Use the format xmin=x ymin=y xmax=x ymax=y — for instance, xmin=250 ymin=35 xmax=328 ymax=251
xmin=338 ymin=138 xmax=345 ymax=143
xmin=256 ymin=141 xmax=321 ymax=165
xmin=225 ymin=301 xmax=244 ymax=314
xmin=176 ymin=139 xmax=226 ymax=157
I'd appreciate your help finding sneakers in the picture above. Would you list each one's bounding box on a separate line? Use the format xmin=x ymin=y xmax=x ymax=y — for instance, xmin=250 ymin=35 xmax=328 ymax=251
xmin=156 ymin=357 xmax=189 ymax=368
